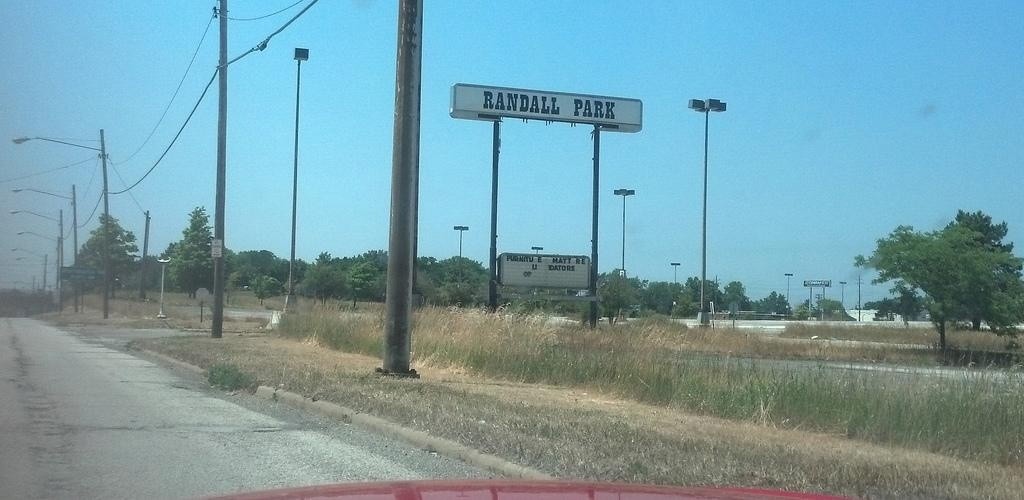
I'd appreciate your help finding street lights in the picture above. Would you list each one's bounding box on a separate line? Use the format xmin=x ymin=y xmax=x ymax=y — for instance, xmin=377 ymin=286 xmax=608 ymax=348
xmin=9 ymin=128 xmax=112 ymax=318
xmin=531 ymin=246 xmax=543 ymax=295
xmin=784 ymin=273 xmax=793 ymax=302
xmin=688 ymin=99 xmax=726 ymax=311
xmin=840 ymin=282 xmax=847 ymax=303
xmin=671 ymin=262 xmax=681 ymax=284
xmin=454 ymin=226 xmax=468 ymax=287
xmin=614 ymin=188 xmax=635 ymax=280
xmin=283 ymin=48 xmax=309 ymax=314
